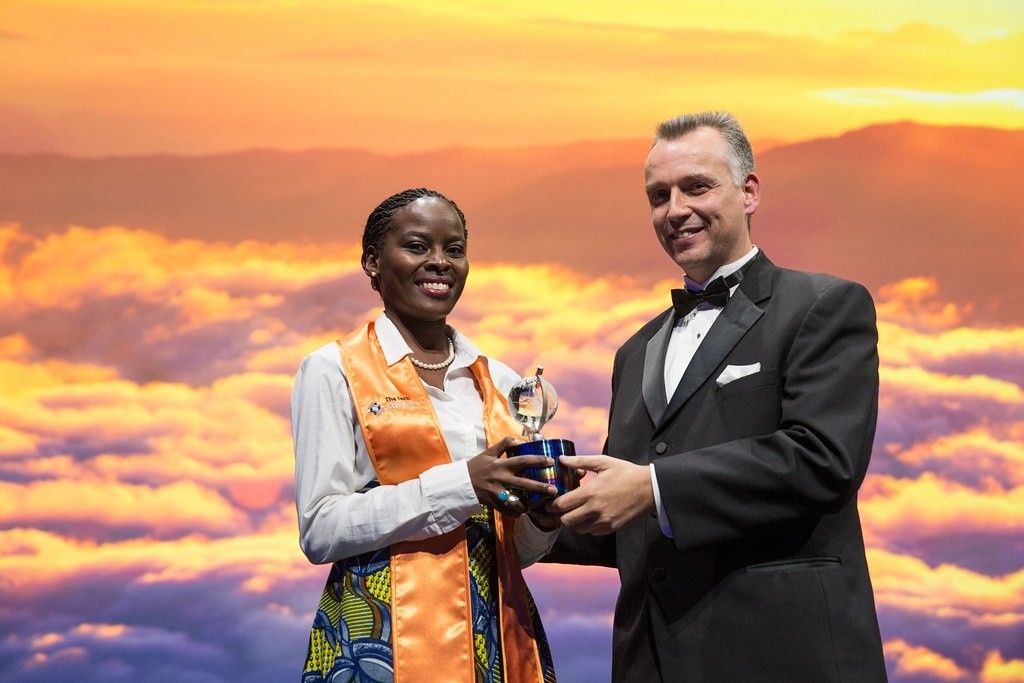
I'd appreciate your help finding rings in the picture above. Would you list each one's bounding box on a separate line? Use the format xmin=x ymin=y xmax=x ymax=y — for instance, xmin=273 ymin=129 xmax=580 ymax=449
xmin=497 ymin=490 xmax=510 ymax=502
xmin=504 ymin=495 xmax=520 ymax=507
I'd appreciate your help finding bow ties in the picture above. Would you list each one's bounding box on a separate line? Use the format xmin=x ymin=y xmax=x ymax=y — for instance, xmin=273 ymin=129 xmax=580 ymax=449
xmin=671 ymin=275 xmax=730 ymax=318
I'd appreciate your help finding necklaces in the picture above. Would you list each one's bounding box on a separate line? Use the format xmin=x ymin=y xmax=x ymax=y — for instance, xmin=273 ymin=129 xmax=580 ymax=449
xmin=407 ymin=335 xmax=455 ymax=369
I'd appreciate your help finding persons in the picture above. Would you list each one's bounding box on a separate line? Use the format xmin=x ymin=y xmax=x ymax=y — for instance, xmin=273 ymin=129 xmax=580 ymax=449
xmin=291 ymin=187 xmax=588 ymax=683
xmin=536 ymin=110 xmax=889 ymax=683
